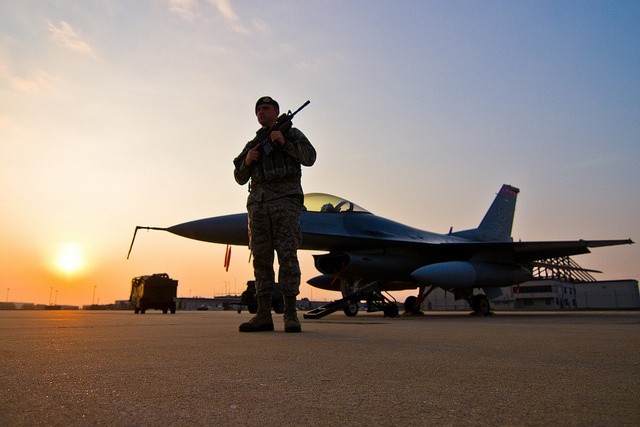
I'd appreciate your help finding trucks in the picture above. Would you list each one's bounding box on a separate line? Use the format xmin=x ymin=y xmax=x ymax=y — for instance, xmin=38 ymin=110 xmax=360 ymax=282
xmin=243 ymin=279 xmax=285 ymax=315
xmin=129 ymin=273 xmax=179 ymax=314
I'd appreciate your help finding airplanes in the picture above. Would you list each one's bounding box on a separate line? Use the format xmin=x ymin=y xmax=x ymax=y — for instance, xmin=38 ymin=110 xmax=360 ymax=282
xmin=126 ymin=183 xmax=634 ymax=317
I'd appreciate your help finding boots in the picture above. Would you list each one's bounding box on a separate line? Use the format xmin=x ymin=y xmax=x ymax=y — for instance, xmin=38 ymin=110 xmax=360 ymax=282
xmin=239 ymin=292 xmax=273 ymax=332
xmin=283 ymin=295 xmax=301 ymax=332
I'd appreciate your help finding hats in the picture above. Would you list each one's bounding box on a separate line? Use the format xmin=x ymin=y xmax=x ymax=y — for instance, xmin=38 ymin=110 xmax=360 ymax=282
xmin=256 ymin=97 xmax=279 ymax=115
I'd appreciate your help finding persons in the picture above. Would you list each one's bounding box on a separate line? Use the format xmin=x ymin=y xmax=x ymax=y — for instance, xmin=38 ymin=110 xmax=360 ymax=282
xmin=233 ymin=95 xmax=317 ymax=333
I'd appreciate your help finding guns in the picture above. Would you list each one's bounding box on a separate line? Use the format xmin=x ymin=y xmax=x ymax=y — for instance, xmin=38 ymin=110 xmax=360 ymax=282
xmin=233 ymin=100 xmax=310 ymax=167
